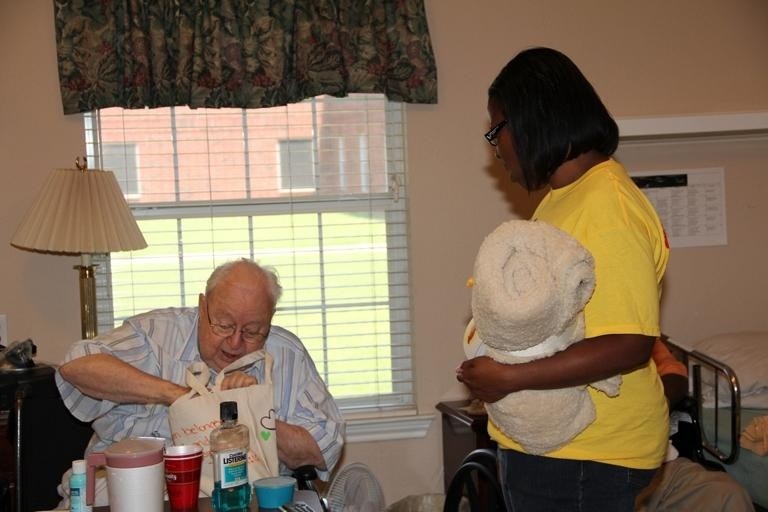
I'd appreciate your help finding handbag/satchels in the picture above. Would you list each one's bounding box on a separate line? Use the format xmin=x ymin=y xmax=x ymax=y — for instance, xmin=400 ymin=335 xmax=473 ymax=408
xmin=167 ymin=349 xmax=282 ymax=500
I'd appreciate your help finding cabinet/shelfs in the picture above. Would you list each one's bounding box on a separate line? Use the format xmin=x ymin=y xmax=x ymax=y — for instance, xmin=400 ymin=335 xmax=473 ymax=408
xmin=0 ymin=365 xmax=61 ymax=512
xmin=435 ymin=399 xmax=498 ymax=511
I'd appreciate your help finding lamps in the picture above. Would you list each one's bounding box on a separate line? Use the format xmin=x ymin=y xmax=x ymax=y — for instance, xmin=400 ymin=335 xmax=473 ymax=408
xmin=11 ymin=153 xmax=148 ymax=341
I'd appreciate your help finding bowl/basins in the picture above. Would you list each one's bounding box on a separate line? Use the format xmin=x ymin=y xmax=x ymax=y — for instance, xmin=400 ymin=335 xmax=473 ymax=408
xmin=104 ymin=437 xmax=164 ymax=512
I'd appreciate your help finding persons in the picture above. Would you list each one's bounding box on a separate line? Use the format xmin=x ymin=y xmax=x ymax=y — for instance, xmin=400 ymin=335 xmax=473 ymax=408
xmin=456 ymin=47 xmax=669 ymax=512
xmin=55 ymin=259 xmax=346 ymax=491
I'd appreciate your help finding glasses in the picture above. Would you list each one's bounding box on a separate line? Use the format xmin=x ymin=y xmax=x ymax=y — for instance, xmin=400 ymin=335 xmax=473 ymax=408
xmin=206 ymin=299 xmax=272 ymax=345
xmin=484 ymin=118 xmax=510 ymax=146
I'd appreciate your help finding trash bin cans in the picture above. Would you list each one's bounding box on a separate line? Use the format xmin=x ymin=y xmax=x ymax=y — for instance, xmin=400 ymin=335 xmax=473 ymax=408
xmin=384 ymin=493 xmax=471 ymax=512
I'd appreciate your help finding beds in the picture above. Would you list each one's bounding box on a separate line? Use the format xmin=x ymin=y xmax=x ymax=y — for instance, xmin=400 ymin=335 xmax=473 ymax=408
xmin=661 ymin=325 xmax=768 ymax=511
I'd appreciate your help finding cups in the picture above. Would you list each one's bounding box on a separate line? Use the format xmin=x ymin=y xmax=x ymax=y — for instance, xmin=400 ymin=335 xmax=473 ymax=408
xmin=161 ymin=443 xmax=203 ymax=512
xmin=6 ymin=338 xmax=37 ymax=366
xmin=253 ymin=476 xmax=298 ymax=509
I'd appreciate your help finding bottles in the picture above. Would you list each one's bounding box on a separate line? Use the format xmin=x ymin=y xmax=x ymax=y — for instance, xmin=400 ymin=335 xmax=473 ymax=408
xmin=68 ymin=460 xmax=92 ymax=512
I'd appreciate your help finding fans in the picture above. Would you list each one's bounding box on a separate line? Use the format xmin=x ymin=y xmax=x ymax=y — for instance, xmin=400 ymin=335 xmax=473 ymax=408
xmin=326 ymin=461 xmax=386 ymax=512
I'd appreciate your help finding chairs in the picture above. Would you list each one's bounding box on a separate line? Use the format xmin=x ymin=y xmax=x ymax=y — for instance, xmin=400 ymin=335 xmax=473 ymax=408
xmin=13 ymin=382 xmax=96 ymax=511
xmin=443 ymin=446 xmax=510 ymax=511
